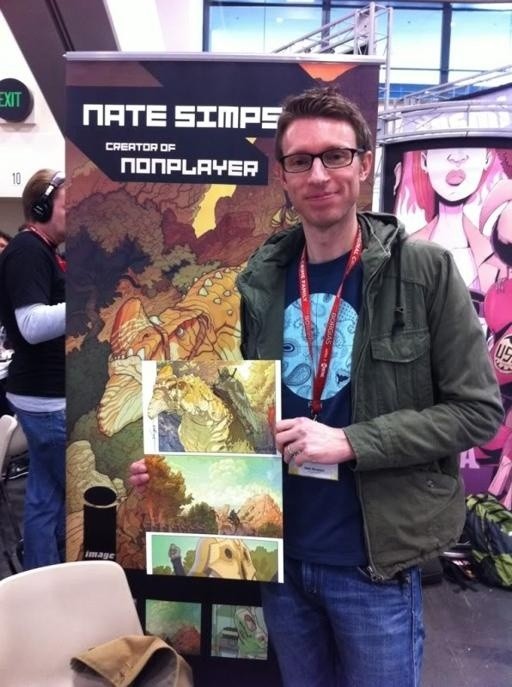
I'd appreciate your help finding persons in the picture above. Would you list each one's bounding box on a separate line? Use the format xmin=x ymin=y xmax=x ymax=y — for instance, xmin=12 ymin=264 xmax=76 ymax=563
xmin=403 ymin=145 xmax=512 ymax=512
xmin=168 ymin=537 xmax=258 ymax=581
xmin=130 ymin=86 xmax=506 ymax=687
xmin=0 ymin=165 xmax=68 ymax=575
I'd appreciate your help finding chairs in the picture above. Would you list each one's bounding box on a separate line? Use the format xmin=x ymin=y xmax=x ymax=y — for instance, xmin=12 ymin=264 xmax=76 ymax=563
xmin=1 ymin=558 xmax=145 ymax=687
xmin=0 ymin=411 xmax=30 ymax=572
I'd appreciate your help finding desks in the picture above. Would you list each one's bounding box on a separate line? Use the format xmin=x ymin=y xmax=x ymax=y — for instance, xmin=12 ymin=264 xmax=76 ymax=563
xmin=0 ymin=356 xmax=13 ymax=385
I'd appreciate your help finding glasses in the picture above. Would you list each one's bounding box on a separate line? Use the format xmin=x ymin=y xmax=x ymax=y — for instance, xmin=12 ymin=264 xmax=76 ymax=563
xmin=277 ymin=147 xmax=365 ymax=174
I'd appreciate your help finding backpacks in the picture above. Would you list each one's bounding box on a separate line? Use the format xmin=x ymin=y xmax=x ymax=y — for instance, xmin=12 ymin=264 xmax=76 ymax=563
xmin=456 ymin=489 xmax=512 ymax=589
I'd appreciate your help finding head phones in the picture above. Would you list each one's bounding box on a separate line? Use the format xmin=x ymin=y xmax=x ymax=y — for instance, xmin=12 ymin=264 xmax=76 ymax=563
xmin=28 ymin=170 xmax=66 ymax=222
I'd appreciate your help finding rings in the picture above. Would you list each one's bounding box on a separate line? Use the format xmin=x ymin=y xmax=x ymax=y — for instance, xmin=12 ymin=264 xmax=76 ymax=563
xmin=287 ymin=445 xmax=294 ymax=457
